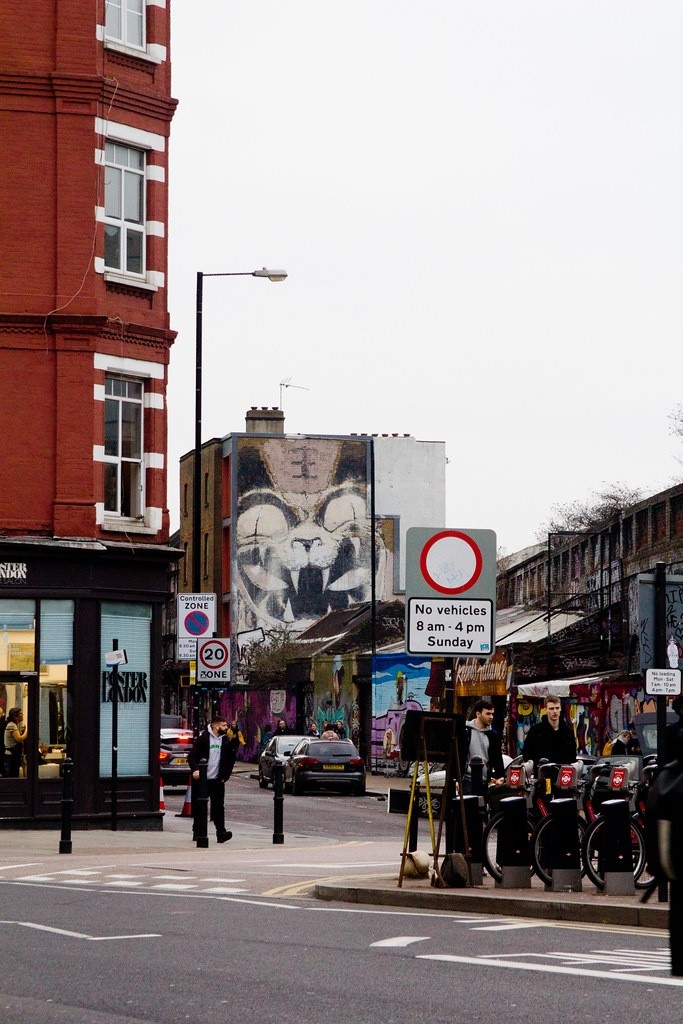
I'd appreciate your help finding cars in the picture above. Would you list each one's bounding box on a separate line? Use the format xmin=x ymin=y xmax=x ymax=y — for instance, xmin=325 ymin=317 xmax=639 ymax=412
xmin=414 ymin=760 xmax=448 ymax=793
xmin=259 ymin=735 xmax=321 ymax=794
xmin=160 ymin=728 xmax=196 ymax=785
xmin=490 ymin=755 xmax=599 ymax=810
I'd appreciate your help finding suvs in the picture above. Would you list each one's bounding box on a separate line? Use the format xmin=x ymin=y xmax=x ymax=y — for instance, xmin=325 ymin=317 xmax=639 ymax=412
xmin=283 ymin=738 xmax=367 ymax=798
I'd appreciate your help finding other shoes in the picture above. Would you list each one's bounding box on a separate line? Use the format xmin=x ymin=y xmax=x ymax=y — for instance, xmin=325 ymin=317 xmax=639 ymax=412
xmin=216 ymin=829 xmax=232 ymax=843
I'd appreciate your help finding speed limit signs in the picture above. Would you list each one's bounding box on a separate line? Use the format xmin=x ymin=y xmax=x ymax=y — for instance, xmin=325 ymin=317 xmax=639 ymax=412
xmin=200 ymin=640 xmax=228 ymax=669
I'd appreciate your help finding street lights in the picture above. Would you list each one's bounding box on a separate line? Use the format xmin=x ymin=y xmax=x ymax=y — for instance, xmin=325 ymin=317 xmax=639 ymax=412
xmin=546 ymin=531 xmax=577 ymax=679
xmin=192 ymin=268 xmax=289 ymax=592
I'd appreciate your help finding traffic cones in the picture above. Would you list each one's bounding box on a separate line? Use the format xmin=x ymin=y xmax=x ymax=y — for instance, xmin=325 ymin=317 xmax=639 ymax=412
xmin=159 ymin=777 xmax=165 ymax=810
xmin=175 ymin=775 xmax=192 ymax=818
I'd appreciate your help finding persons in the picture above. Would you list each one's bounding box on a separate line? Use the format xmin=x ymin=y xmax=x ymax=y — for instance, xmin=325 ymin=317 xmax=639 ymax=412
xmin=305 ymin=723 xmax=320 ymax=738
xmin=273 ymin=721 xmax=291 ymax=735
xmin=665 ymin=695 xmax=683 ymax=762
xmin=4 ymin=708 xmax=27 ymax=777
xmin=602 ymin=722 xmax=643 ymax=756
xmin=521 ymin=696 xmax=577 ymax=778
xmin=188 ymin=716 xmax=232 ymax=844
xmin=462 ymin=700 xmax=506 ymax=787
xmin=320 ymin=719 xmax=346 ymax=739
xmin=227 ymin=721 xmax=246 ymax=766
xmin=264 ymin=725 xmax=272 ymax=744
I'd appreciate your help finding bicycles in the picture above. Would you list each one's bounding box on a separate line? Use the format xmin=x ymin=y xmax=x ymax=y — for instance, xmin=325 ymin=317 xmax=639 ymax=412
xmin=480 ymin=755 xmax=662 ymax=891
